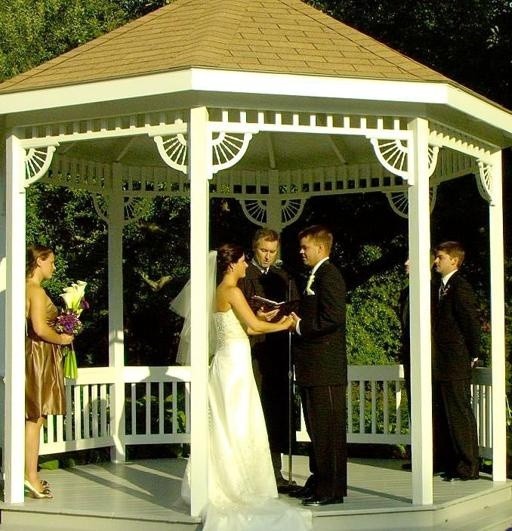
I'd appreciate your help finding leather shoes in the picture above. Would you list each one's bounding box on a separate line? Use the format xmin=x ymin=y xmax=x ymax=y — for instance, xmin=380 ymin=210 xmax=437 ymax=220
xmin=302 ymin=492 xmax=345 ymax=505
xmin=440 ymin=471 xmax=453 ymax=477
xmin=402 ymin=463 xmax=412 ymax=471
xmin=442 ymin=471 xmax=479 ymax=482
xmin=290 ymin=485 xmax=315 ymax=498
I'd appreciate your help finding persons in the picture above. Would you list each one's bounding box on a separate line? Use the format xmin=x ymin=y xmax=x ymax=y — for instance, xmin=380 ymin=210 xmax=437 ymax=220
xmin=21 ymin=247 xmax=75 ymax=500
xmin=175 ymin=244 xmax=294 ymax=509
xmin=395 ymin=251 xmax=434 ymax=470
xmin=433 ymin=240 xmax=482 ymax=480
xmin=236 ymin=227 xmax=307 ymax=494
xmin=287 ymin=226 xmax=349 ymax=506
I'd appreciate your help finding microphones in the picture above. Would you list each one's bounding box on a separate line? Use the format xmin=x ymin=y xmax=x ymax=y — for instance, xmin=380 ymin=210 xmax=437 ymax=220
xmin=276 ymin=260 xmax=295 ymax=278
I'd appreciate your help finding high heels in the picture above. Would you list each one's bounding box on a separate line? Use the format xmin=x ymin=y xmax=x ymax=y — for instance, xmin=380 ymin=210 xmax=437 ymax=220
xmin=24 ymin=480 xmax=53 ymax=498
xmin=41 ymin=478 xmax=49 ymax=486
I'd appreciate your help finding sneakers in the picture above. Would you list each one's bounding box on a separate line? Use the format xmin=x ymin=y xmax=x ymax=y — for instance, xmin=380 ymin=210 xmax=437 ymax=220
xmin=277 ymin=478 xmax=297 ymax=488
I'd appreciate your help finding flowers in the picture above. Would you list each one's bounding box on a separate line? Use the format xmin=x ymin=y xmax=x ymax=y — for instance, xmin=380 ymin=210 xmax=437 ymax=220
xmin=51 ymin=280 xmax=90 ymax=378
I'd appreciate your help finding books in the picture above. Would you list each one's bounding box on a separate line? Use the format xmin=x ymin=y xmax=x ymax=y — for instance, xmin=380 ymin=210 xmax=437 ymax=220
xmin=251 ymin=295 xmax=301 ymax=321
